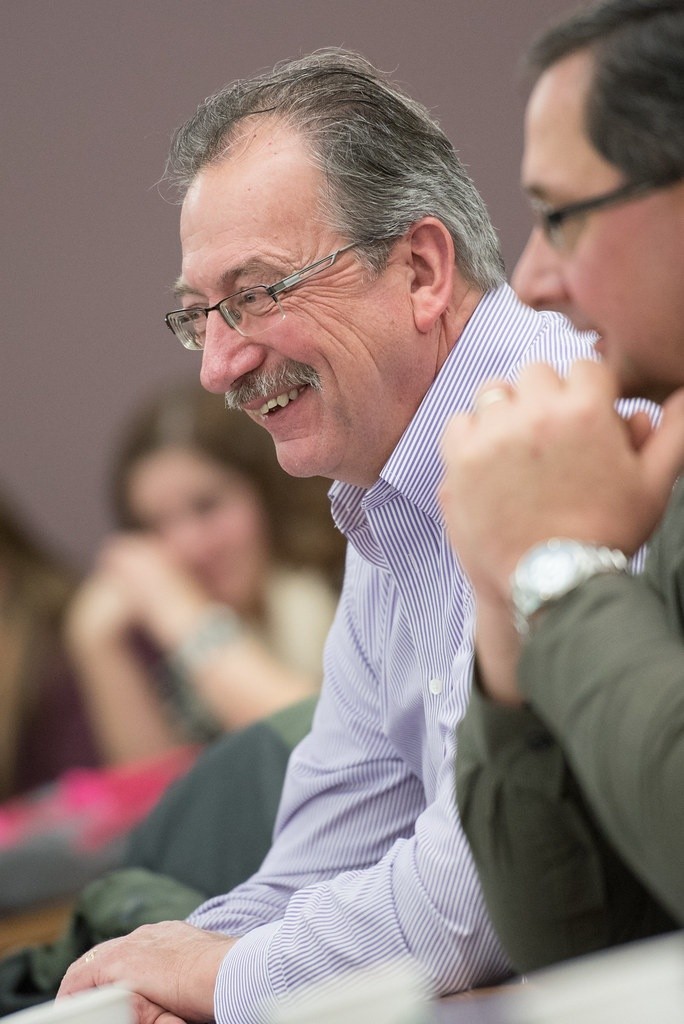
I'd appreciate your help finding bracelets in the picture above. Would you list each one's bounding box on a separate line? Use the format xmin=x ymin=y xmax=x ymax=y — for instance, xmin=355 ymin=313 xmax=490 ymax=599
xmin=167 ymin=603 xmax=238 ymax=677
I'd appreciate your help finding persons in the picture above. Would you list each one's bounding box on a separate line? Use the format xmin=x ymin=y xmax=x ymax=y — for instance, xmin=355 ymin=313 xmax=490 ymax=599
xmin=58 ymin=47 xmax=664 ymax=1024
xmin=2 ymin=384 xmax=343 ymax=805
xmin=436 ymin=0 xmax=684 ymax=971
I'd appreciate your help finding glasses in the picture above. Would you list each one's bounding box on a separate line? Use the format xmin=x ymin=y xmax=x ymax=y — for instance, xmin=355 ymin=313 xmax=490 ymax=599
xmin=532 ymin=170 xmax=684 ymax=250
xmin=164 ymin=236 xmax=402 ymax=351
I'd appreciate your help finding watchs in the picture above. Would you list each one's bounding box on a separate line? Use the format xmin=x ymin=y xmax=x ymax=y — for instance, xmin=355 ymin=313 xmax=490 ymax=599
xmin=502 ymin=540 xmax=632 ymax=637
